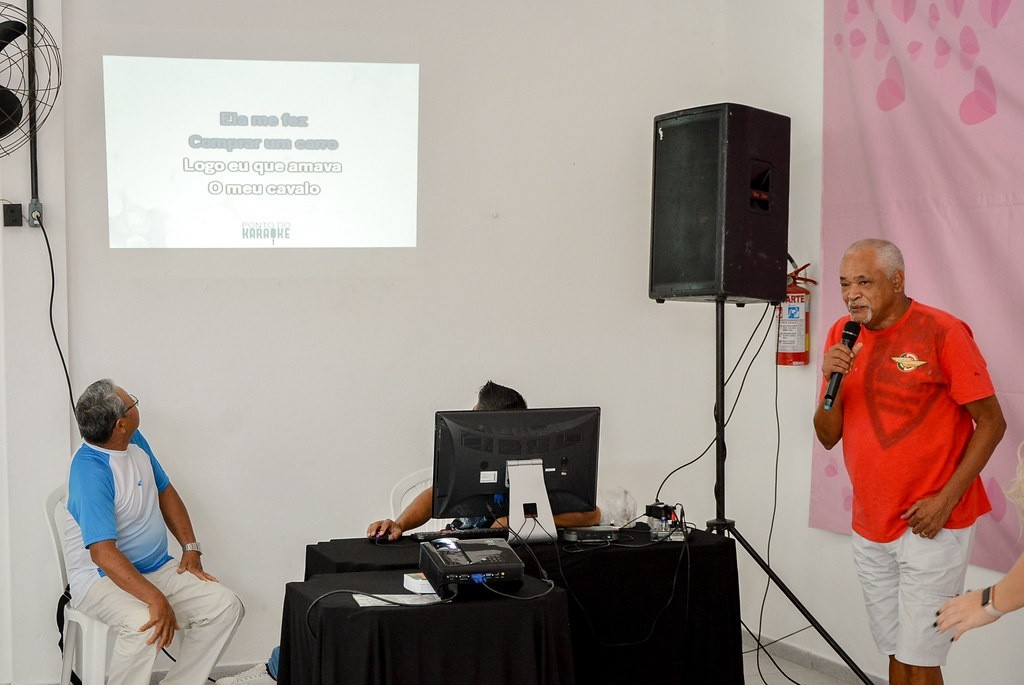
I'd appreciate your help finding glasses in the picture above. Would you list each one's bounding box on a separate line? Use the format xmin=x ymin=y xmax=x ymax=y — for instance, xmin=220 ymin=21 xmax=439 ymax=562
xmin=113 ymin=393 xmax=139 ymax=428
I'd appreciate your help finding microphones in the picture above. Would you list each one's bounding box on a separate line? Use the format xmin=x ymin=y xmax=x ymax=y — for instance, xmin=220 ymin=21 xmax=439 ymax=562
xmin=824 ymin=321 xmax=862 ymax=411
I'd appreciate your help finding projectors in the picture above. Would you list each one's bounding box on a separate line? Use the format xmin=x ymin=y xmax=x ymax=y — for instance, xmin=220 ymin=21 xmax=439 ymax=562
xmin=418 ymin=539 xmax=525 ymax=595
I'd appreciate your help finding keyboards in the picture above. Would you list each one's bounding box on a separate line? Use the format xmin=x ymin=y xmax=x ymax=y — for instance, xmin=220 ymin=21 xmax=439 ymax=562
xmin=411 ymin=524 xmax=566 ymax=543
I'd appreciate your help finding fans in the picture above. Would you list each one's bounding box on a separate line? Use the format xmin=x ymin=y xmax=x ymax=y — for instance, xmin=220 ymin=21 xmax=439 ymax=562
xmin=0 ymin=3 xmax=62 ymax=159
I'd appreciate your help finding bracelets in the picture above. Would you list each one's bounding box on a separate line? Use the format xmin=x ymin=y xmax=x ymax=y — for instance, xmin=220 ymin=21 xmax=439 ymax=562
xmin=183 ymin=543 xmax=202 ymax=556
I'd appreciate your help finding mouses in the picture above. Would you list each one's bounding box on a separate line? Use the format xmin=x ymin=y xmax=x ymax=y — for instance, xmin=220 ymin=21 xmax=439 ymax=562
xmin=368 ymin=528 xmax=392 ymax=543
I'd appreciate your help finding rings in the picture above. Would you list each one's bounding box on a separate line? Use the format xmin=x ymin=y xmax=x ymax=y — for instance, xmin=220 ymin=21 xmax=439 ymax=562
xmin=923 ymin=531 xmax=928 ymax=537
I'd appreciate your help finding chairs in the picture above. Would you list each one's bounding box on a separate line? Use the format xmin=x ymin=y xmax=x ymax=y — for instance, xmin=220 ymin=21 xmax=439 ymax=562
xmin=44 ymin=483 xmax=220 ymax=685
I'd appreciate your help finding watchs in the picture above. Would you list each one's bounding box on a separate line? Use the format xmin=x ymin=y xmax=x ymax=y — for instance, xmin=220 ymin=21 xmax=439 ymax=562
xmin=981 ymin=585 xmax=1005 ymax=617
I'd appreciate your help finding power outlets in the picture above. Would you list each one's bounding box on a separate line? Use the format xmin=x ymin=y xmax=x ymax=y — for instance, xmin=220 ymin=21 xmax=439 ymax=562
xmin=26 ymin=202 xmax=44 ymax=228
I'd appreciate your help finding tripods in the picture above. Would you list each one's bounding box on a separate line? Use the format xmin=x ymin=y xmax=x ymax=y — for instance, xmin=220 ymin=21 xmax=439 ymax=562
xmin=702 ymin=299 xmax=875 ymax=685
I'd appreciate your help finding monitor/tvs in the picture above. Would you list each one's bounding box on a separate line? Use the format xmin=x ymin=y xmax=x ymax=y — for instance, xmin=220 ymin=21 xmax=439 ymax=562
xmin=432 ymin=405 xmax=601 ymax=542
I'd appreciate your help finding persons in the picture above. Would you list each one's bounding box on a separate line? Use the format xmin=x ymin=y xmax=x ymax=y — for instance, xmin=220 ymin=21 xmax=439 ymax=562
xmin=216 ymin=381 xmax=601 ymax=685
xmin=65 ymin=378 xmax=243 ymax=685
xmin=813 ymin=239 xmax=1007 ymax=684
xmin=932 ymin=553 xmax=1024 ymax=643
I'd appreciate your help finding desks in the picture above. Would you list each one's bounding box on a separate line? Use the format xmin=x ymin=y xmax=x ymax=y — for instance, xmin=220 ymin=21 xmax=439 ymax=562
xmin=276 ymin=521 xmax=746 ymax=685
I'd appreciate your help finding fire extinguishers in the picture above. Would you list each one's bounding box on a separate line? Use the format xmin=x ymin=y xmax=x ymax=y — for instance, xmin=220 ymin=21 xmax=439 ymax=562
xmin=773 ymin=262 xmax=817 ymax=370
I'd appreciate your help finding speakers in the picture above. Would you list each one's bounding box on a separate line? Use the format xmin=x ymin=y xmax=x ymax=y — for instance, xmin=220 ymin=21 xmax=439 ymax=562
xmin=649 ymin=103 xmax=791 ymax=307
